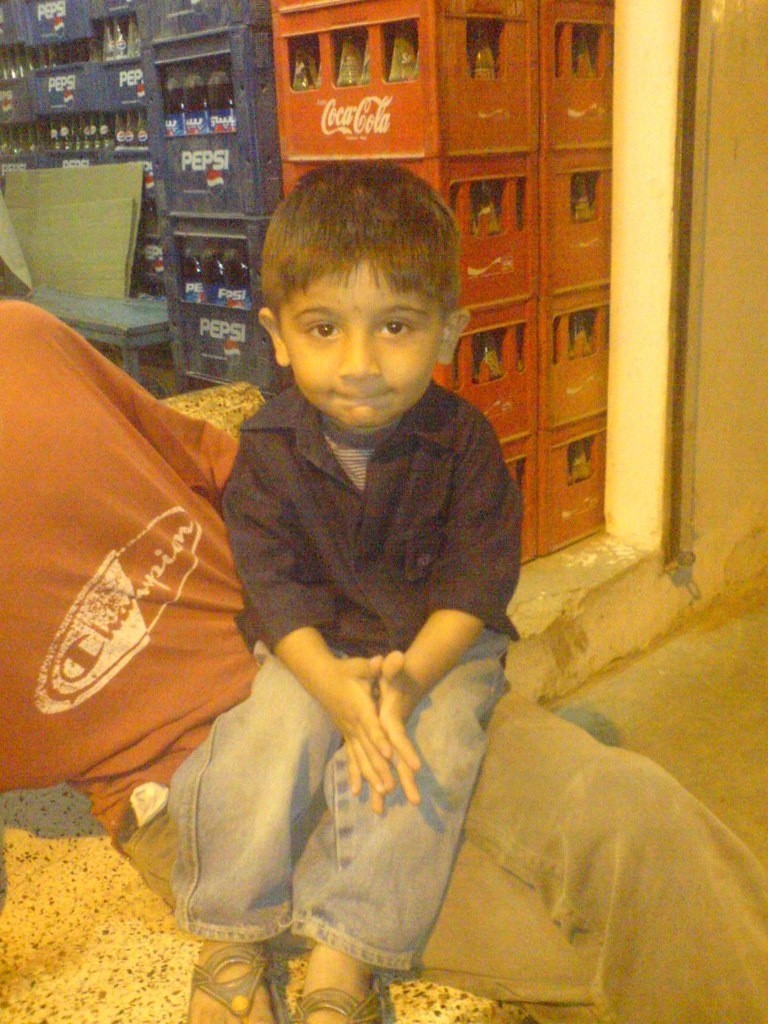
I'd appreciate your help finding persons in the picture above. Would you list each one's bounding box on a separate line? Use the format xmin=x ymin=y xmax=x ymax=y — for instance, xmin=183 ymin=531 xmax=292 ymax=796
xmin=168 ymin=164 xmax=523 ymax=1024
xmin=0 ymin=295 xmax=768 ymax=1024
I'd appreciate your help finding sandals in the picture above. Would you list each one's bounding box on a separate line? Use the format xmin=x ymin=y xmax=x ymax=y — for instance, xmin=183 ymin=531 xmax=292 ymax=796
xmin=188 ymin=945 xmax=284 ymax=1024
xmin=298 ymin=973 xmax=382 ymax=1024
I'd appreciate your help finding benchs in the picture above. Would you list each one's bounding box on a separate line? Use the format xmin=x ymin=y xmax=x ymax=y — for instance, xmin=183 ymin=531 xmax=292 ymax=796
xmin=28 ymin=286 xmax=169 ymax=388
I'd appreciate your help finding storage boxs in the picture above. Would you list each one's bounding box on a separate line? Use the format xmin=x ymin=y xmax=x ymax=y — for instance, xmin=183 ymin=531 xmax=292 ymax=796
xmin=1 ymin=0 xmax=618 ymax=562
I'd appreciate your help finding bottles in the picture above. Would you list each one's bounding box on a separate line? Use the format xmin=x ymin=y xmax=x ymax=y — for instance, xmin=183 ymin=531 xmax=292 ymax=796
xmin=0 ymin=0 xmax=595 ymax=485
